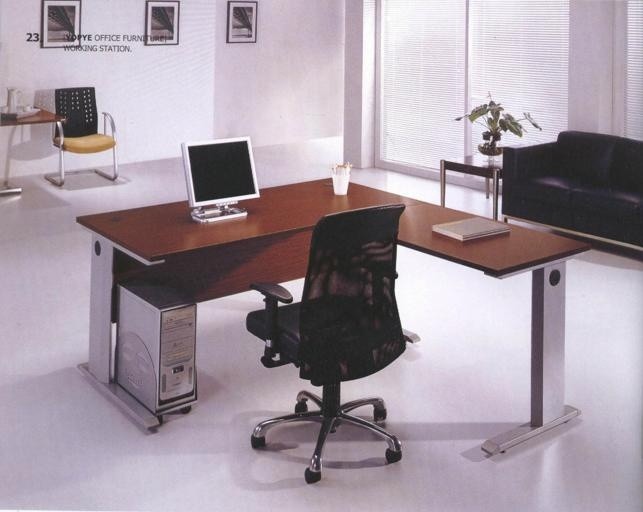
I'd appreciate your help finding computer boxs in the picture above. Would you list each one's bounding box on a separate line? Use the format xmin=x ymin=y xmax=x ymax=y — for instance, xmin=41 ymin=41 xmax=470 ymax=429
xmin=117 ymin=283 xmax=197 ymax=417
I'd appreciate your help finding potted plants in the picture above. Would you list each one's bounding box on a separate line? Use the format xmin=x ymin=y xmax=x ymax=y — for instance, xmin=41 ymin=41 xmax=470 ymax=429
xmin=453 ymin=92 xmax=542 ymax=156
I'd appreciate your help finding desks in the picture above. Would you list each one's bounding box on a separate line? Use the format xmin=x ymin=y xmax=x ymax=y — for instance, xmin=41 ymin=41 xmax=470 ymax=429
xmin=440 ymin=147 xmax=514 ymax=222
xmin=75 ymin=178 xmax=592 ymax=459
xmin=1 ymin=107 xmax=68 ymax=195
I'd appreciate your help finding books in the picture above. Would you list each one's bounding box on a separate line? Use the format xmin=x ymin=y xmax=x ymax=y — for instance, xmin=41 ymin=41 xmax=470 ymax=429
xmin=430 ymin=216 xmax=511 ymax=242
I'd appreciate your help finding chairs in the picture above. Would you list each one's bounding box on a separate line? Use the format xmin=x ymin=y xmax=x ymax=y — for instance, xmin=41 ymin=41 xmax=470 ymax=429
xmin=246 ymin=204 xmax=421 ymax=484
xmin=45 ymin=87 xmax=118 ymax=186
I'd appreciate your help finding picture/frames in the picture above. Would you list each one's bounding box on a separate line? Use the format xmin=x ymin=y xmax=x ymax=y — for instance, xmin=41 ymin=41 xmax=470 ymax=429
xmin=226 ymin=1 xmax=258 ymax=44
xmin=144 ymin=1 xmax=180 ymax=45
xmin=41 ymin=0 xmax=82 ymax=48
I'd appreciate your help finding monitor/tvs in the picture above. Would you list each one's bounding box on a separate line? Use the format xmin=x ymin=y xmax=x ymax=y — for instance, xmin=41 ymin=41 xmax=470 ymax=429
xmin=181 ymin=137 xmax=260 ymax=223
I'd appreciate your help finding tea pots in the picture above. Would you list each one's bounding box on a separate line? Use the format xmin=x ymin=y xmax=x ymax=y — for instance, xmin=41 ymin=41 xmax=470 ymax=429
xmin=7 ymin=88 xmax=23 ymax=114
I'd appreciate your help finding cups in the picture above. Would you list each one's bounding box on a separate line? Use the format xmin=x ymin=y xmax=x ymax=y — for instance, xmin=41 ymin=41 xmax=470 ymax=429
xmin=331 ymin=167 xmax=350 ymax=195
xmin=23 ymin=105 xmax=32 ymax=112
xmin=0 ymin=106 xmax=8 ymax=114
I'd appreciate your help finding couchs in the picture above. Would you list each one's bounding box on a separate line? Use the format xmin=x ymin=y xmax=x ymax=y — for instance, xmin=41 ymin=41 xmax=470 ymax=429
xmin=502 ymin=130 xmax=643 ymax=252
